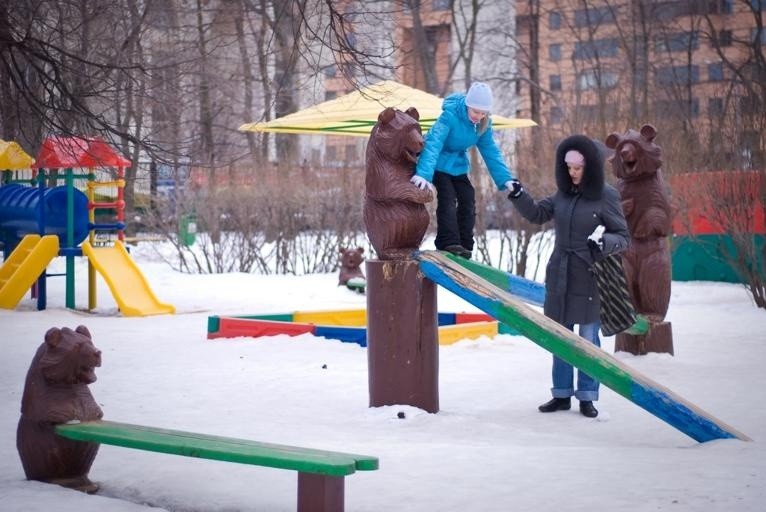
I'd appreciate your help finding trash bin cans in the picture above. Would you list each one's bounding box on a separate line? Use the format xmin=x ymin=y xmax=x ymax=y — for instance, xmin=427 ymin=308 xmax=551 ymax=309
xmin=178 ymin=213 xmax=197 ymax=245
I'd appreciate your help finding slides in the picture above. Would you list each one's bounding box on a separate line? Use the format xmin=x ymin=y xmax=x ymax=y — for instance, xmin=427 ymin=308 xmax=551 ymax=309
xmin=81 ymin=240 xmax=175 ymax=316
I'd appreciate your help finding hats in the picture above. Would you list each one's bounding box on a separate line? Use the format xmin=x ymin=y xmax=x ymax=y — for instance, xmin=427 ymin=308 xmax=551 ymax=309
xmin=563 ymin=148 xmax=584 ymax=166
xmin=464 ymin=82 xmax=493 ymax=111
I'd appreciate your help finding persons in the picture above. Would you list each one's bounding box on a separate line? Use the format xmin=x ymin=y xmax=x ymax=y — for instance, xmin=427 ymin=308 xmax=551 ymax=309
xmin=504 ymin=131 xmax=632 ymax=419
xmin=410 ymin=80 xmax=513 ymax=254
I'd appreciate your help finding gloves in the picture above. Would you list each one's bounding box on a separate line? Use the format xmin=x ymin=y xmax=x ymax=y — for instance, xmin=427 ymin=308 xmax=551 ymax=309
xmin=409 ymin=173 xmax=432 ymax=191
xmin=586 ymin=238 xmax=605 ymax=254
xmin=504 ymin=177 xmax=522 ymax=200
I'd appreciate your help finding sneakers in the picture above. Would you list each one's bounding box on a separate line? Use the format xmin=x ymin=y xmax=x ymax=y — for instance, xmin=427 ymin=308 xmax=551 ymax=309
xmin=444 ymin=244 xmax=471 ymax=259
xmin=579 ymin=400 xmax=597 ymax=417
xmin=538 ymin=397 xmax=571 ymax=412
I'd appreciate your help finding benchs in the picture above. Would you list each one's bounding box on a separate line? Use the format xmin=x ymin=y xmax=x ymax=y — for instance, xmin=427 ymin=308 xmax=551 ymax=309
xmin=51 ymin=419 xmax=379 ymax=511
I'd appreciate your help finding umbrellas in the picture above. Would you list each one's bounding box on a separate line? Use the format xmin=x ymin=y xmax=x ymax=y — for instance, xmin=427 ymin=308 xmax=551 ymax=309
xmin=234 ymin=70 xmax=539 ymax=139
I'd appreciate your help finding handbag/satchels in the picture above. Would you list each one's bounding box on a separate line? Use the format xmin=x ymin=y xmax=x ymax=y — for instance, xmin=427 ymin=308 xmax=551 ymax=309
xmin=591 ymin=254 xmax=637 ymax=336
